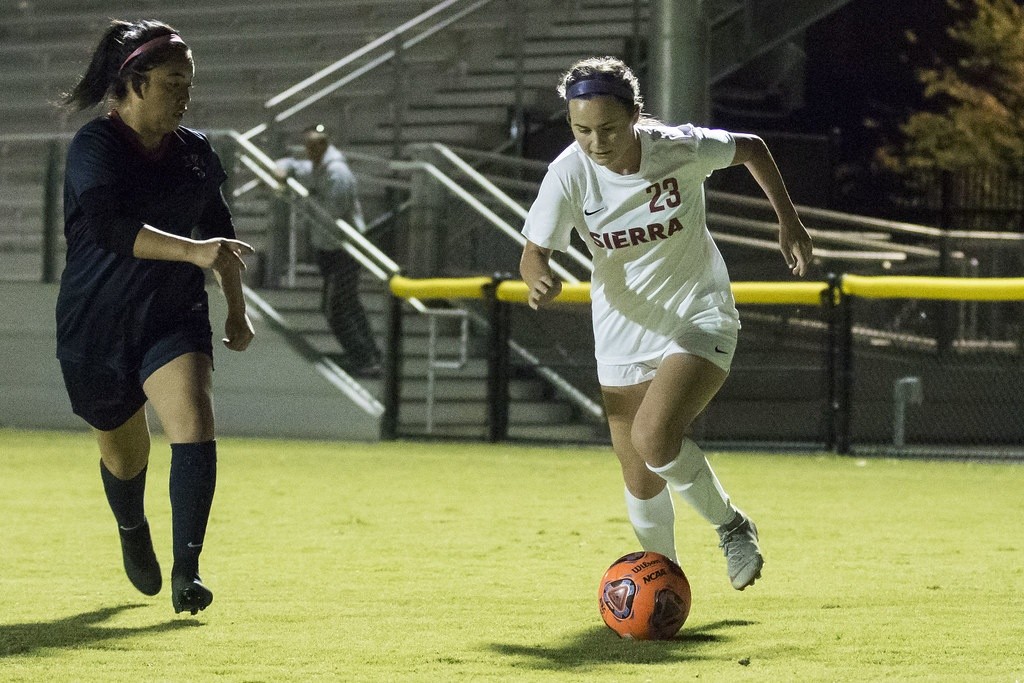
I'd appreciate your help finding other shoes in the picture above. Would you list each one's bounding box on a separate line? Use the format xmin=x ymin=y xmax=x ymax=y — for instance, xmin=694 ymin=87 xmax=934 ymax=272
xmin=329 ymin=347 xmax=384 ymax=379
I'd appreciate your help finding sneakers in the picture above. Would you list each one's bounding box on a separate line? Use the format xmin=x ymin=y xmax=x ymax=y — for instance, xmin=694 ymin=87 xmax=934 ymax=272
xmin=116 ymin=513 xmax=162 ymax=597
xmin=170 ymin=562 xmax=213 ymax=615
xmin=715 ymin=509 xmax=765 ymax=593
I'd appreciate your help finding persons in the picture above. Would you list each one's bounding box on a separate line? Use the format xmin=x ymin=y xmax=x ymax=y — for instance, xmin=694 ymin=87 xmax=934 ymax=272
xmin=50 ymin=17 xmax=257 ymax=616
xmin=274 ymin=122 xmax=387 ymax=380
xmin=518 ymin=56 xmax=815 ymax=593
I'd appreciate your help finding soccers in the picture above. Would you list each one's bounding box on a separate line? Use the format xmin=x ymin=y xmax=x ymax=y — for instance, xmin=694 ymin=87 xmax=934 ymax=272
xmin=595 ymin=548 xmax=693 ymax=643
